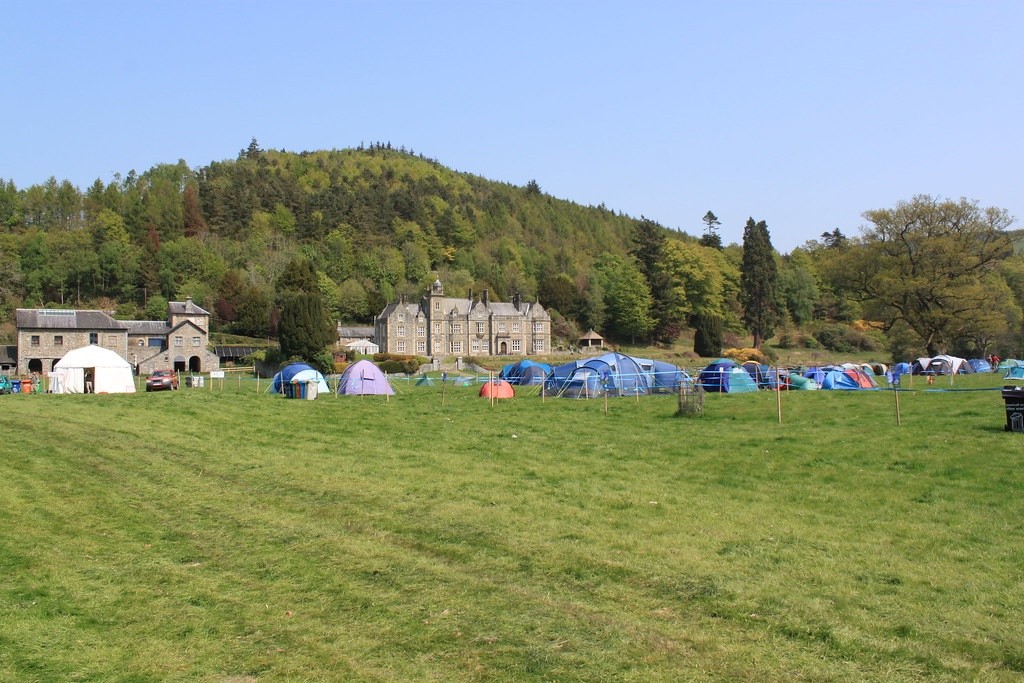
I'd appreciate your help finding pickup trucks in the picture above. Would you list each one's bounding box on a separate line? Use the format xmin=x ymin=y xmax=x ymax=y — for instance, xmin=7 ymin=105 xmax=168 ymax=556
xmin=146 ymin=370 xmax=179 ymax=391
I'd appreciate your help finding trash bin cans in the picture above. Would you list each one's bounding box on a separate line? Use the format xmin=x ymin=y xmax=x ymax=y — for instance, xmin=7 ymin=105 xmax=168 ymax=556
xmin=1000 ymin=385 xmax=1024 ymax=433
xmin=21 ymin=379 xmax=32 ymax=394
xmin=334 ymin=351 xmax=346 ymax=363
xmin=10 ymin=380 xmax=22 ymax=394
xmin=31 ymin=380 xmax=42 ymax=394
xmin=283 ymin=379 xmax=320 ymax=400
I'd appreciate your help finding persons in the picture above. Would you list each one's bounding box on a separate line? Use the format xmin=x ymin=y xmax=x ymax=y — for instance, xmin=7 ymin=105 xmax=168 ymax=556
xmin=441 ymin=371 xmax=447 ymax=382
xmin=29 ymin=372 xmax=39 ymax=395
xmin=85 ymin=370 xmax=93 ymax=394
xmin=992 ymin=354 xmax=1000 ymax=370
xmin=987 ymin=354 xmax=992 ymax=365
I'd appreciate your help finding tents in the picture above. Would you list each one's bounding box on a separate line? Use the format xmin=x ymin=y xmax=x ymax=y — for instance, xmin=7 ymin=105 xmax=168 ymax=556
xmin=416 ymin=373 xmax=435 ymax=386
xmin=453 ymin=378 xmax=473 ymax=387
xmin=337 ymin=360 xmax=395 ymax=395
xmin=268 ymin=363 xmax=329 ymax=394
xmin=479 ymin=353 xmax=1024 ymax=398
xmin=53 ymin=345 xmax=136 ymax=393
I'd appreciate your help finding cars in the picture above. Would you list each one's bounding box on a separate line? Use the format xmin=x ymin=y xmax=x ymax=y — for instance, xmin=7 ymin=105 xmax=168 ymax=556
xmin=0 ymin=376 xmax=12 ymax=394
